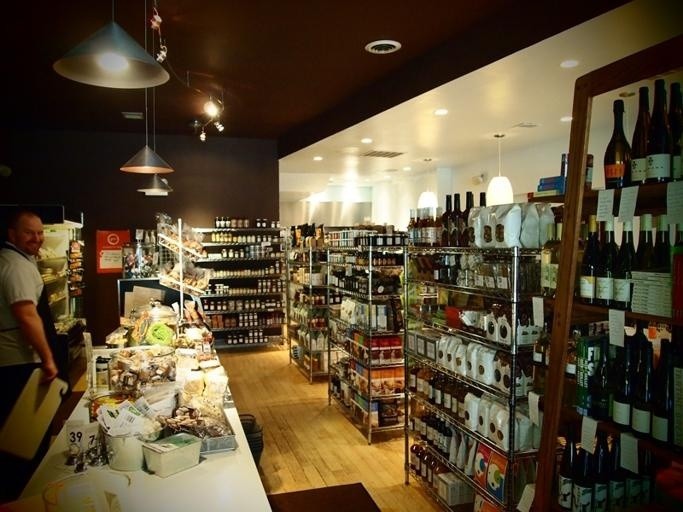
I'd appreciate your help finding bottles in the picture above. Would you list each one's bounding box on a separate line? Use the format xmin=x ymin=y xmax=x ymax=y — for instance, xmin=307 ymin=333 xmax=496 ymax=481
xmin=150 ymin=231 xmax=156 ymax=243
xmin=556 ymin=76 xmax=682 ymax=512
xmin=404 ymin=191 xmax=566 ymax=512
xmin=280 ymin=223 xmax=408 ymax=432
xmin=95 ymin=358 xmax=110 ymax=393
xmin=201 ymin=216 xmax=284 ymax=345
xmin=144 ymin=231 xmax=150 ymax=245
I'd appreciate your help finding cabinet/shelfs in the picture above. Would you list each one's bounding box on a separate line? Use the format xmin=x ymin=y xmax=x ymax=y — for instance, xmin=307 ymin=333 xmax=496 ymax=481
xmin=532 ymin=42 xmax=681 ymax=511
xmin=116 ymin=213 xmax=283 ymax=350
xmin=0 ymin=204 xmax=86 ymax=335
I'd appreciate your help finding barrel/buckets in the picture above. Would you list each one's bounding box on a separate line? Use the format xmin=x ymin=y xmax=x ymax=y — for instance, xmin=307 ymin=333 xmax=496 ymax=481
xmin=463 ymin=391 xmax=543 ymax=453
xmin=435 ymin=336 xmax=494 ymax=383
xmin=101 ymin=422 xmax=167 ymax=473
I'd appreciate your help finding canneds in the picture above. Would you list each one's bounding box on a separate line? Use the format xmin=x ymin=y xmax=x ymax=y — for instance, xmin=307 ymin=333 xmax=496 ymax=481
xmin=359 ymin=233 xmax=409 ymax=246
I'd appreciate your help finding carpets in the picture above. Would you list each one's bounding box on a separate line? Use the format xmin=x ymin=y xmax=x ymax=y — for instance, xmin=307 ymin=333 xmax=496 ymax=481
xmin=266 ymin=482 xmax=382 ymax=512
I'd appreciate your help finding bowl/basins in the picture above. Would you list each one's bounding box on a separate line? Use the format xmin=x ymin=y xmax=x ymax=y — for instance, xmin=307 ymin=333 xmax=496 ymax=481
xmin=111 ymin=346 xmax=179 ymax=398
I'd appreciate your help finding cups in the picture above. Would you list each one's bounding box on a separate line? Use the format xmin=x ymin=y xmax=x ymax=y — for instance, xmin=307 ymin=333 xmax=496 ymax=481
xmin=43 ymin=469 xmax=131 ymax=511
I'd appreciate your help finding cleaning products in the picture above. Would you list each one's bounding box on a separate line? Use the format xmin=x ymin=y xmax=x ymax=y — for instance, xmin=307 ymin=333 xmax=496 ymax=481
xmin=445 ymin=423 xmax=478 ymax=477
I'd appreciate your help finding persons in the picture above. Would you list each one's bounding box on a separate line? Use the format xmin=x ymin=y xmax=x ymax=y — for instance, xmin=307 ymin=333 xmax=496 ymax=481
xmin=0 ymin=207 xmax=73 ymax=504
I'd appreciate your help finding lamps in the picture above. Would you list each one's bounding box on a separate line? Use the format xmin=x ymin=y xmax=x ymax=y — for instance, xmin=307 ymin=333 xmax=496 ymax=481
xmin=416 ymin=158 xmax=439 ymax=209
xmin=48 ymin=1 xmax=170 ymax=89
xmin=485 ymin=134 xmax=514 ymax=207
xmin=119 ymin=1 xmax=175 ymax=198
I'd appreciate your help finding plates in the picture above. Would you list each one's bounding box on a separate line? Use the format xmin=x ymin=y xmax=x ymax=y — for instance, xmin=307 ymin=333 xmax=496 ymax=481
xmin=88 ymin=393 xmax=137 ymax=420
